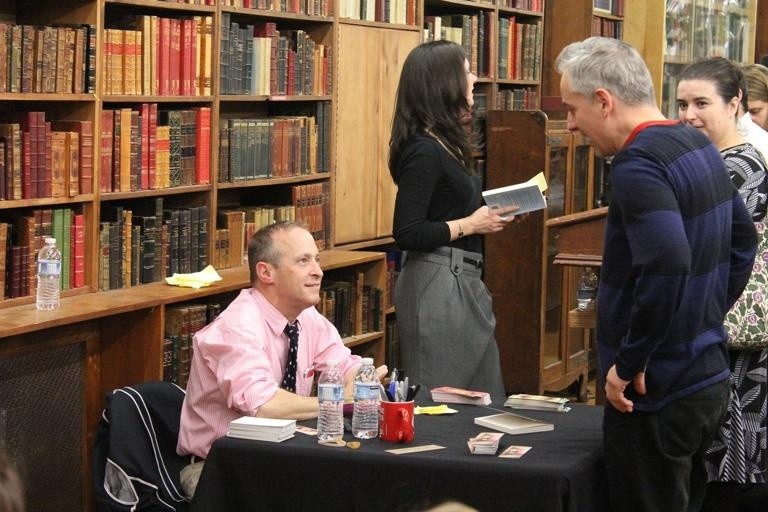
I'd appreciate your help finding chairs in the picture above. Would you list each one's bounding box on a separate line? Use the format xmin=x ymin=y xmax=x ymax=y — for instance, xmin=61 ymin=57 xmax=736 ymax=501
xmin=97 ymin=382 xmax=190 ymax=512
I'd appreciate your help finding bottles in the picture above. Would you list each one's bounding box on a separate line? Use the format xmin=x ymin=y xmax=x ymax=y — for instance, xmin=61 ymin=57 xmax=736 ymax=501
xmin=36 ymin=237 xmax=62 ymax=311
xmin=351 ymin=358 xmax=380 ymax=439
xmin=316 ymin=358 xmax=345 ymax=440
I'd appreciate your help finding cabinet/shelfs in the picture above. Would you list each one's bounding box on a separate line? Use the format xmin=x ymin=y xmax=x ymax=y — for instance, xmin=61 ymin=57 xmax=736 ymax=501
xmin=0 ymin=0 xmax=389 ymax=512
xmin=480 ymin=110 xmax=611 ymax=401
xmin=540 ymin=7 xmax=623 ymax=122
xmin=330 ymin=17 xmax=423 ymax=252
xmin=422 ymin=0 xmax=545 ymax=167
xmin=621 ymin=2 xmax=759 ymax=119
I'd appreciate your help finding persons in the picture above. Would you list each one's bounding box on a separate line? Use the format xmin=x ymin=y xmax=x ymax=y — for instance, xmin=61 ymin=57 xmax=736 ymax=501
xmin=552 ymin=35 xmax=760 ymax=512
xmin=386 ymin=40 xmax=509 ymax=403
xmin=675 ymin=56 xmax=764 ymax=511
xmin=176 ymin=220 xmax=364 ymax=505
xmin=741 ymin=63 xmax=764 ymax=132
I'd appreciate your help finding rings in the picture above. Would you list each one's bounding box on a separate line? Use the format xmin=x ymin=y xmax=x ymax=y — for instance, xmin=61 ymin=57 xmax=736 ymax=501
xmin=498 ymin=216 xmax=508 ymax=224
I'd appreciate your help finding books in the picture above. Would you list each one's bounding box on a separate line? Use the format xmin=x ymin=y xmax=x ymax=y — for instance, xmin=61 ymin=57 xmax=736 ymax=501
xmin=214 ymin=183 xmax=327 ymax=270
xmin=101 ymin=12 xmax=213 ymax=98
xmin=424 ymin=5 xmax=541 ymax=83
xmin=99 ymin=196 xmax=209 ymax=291
xmin=502 ymin=386 xmax=572 ymax=415
xmin=161 ymin=302 xmax=227 ymax=390
xmin=220 ymin=101 xmax=329 ymax=182
xmin=100 ymin=103 xmax=210 ymax=191
xmin=474 ymin=403 xmax=555 ymax=438
xmin=496 ymin=86 xmax=540 ymax=114
xmin=479 ymin=170 xmax=551 ymax=223
xmin=314 ymin=269 xmax=384 ymax=338
xmin=429 ymin=380 xmax=493 ymax=409
xmin=220 ymin=13 xmax=334 ymax=98
xmin=0 ymin=19 xmax=95 ymax=95
xmin=339 ymin=0 xmax=418 ymax=30
xmin=0 ymin=108 xmax=92 ymax=201
xmin=2 ymin=209 xmax=88 ymax=299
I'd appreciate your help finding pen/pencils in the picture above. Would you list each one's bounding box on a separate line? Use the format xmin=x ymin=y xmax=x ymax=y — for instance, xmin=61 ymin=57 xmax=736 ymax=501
xmin=378 ymin=370 xmax=423 ymax=402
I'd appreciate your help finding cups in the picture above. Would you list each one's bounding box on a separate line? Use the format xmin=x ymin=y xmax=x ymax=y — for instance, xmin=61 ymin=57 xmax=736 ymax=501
xmin=379 ymin=401 xmax=415 ymax=443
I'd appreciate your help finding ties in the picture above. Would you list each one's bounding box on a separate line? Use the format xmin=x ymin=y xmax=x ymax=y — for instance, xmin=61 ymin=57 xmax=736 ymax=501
xmin=281 ymin=323 xmax=300 ymax=394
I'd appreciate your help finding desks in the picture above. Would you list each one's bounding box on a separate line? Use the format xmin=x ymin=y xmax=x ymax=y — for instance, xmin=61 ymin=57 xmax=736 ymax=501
xmin=189 ymin=394 xmax=607 ymax=512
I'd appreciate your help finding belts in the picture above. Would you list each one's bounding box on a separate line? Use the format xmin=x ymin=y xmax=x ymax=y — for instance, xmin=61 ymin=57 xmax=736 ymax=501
xmin=435 ymin=249 xmax=484 ymax=268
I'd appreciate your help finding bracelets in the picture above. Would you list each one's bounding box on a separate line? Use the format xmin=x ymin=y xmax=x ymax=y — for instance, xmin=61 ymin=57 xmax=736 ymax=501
xmin=454 ymin=218 xmax=466 ymax=242
